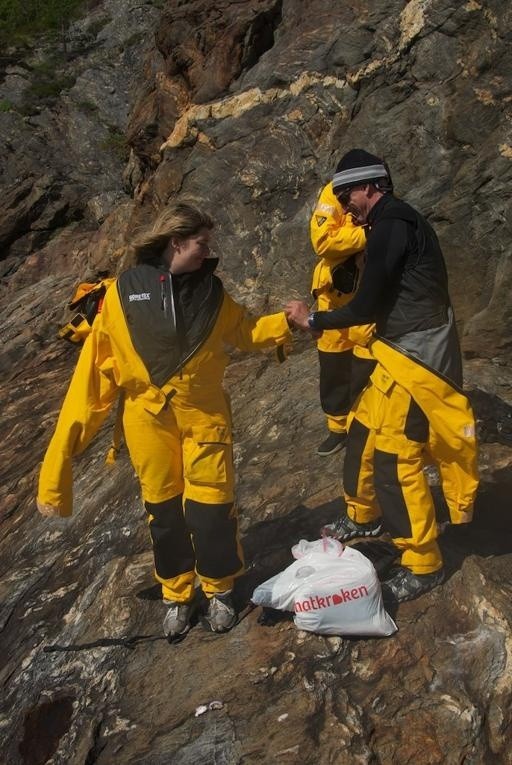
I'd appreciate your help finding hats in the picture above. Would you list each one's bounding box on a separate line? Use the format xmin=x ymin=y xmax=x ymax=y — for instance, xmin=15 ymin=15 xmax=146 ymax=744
xmin=332 ymin=149 xmax=388 ymax=195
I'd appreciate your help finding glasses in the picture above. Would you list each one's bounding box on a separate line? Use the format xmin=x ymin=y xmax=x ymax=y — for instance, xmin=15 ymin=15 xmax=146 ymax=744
xmin=337 ymin=188 xmax=354 ymax=206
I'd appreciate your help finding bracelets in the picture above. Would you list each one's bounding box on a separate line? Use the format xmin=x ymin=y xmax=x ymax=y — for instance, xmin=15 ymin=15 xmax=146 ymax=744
xmin=309 ymin=309 xmax=316 ymax=329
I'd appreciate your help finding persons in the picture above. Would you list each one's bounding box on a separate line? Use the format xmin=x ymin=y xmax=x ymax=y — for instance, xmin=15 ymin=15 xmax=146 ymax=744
xmin=311 ymin=178 xmax=376 ymax=457
xmin=282 ymin=147 xmax=479 ymax=602
xmin=37 ymin=200 xmax=300 ymax=642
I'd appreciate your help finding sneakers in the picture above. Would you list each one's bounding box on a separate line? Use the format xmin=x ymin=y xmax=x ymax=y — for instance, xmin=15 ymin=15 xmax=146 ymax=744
xmin=209 ymin=589 xmax=236 ymax=633
xmin=319 ymin=512 xmax=385 ymax=543
xmin=316 ymin=430 xmax=348 ymax=456
xmin=163 ymin=592 xmax=197 ymax=641
xmin=378 ymin=567 xmax=445 ymax=604
xmin=435 ymin=523 xmax=475 ymax=556
